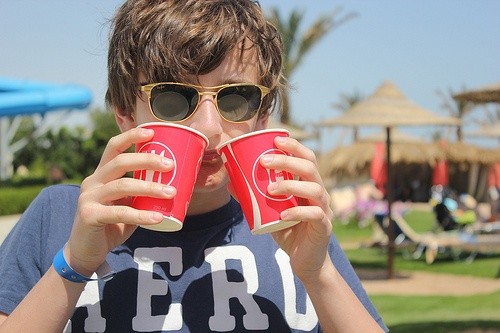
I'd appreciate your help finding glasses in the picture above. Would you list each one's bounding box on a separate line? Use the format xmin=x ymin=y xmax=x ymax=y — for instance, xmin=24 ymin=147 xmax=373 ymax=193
xmin=134 ymin=83 xmax=271 ymax=124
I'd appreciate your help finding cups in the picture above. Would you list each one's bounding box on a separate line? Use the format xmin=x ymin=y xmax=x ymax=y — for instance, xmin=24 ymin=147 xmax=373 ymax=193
xmin=132 ymin=122 xmax=209 ymax=231
xmin=217 ymin=128 xmax=304 ymax=235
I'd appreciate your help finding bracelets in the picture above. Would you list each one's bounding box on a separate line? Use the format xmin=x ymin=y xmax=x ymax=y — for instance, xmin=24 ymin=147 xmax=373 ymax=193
xmin=52 ymin=246 xmax=117 ymax=283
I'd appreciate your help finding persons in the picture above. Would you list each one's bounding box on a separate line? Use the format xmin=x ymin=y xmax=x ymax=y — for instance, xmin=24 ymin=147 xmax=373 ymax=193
xmin=0 ymin=0 xmax=390 ymax=333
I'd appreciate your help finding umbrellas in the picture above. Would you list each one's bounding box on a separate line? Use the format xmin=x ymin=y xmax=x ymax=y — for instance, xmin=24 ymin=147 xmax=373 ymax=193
xmin=318 ymin=129 xmax=500 ymax=203
xmin=321 ymin=78 xmax=466 ymax=279
xmin=470 ymin=120 xmax=500 ymax=138
xmin=265 ymin=118 xmax=309 ymax=140
